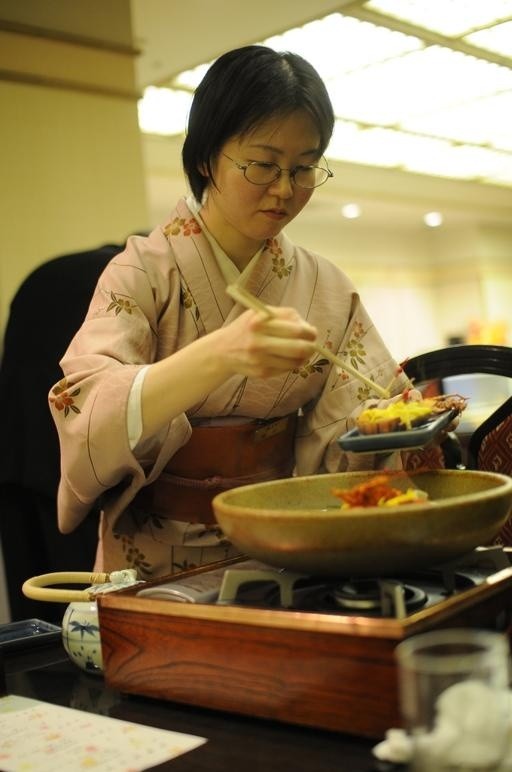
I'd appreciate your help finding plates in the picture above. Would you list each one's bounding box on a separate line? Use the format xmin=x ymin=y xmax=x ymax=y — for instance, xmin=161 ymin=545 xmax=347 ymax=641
xmin=337 ymin=403 xmax=458 ymax=455
xmin=0 ymin=617 xmax=63 ymax=653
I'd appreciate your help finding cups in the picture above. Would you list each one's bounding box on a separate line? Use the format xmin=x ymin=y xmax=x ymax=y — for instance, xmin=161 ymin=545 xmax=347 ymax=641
xmin=395 ymin=631 xmax=510 ymax=772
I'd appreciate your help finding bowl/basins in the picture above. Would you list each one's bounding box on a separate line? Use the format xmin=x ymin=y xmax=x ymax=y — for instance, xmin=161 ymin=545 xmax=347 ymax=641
xmin=212 ymin=468 xmax=512 ymax=577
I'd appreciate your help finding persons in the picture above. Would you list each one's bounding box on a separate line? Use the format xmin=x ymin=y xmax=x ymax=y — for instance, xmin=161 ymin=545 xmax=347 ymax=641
xmin=44 ymin=34 xmax=463 ymax=580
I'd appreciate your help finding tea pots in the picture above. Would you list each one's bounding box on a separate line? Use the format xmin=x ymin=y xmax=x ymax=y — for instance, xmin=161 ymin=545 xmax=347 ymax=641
xmin=21 ymin=570 xmax=147 ymax=676
